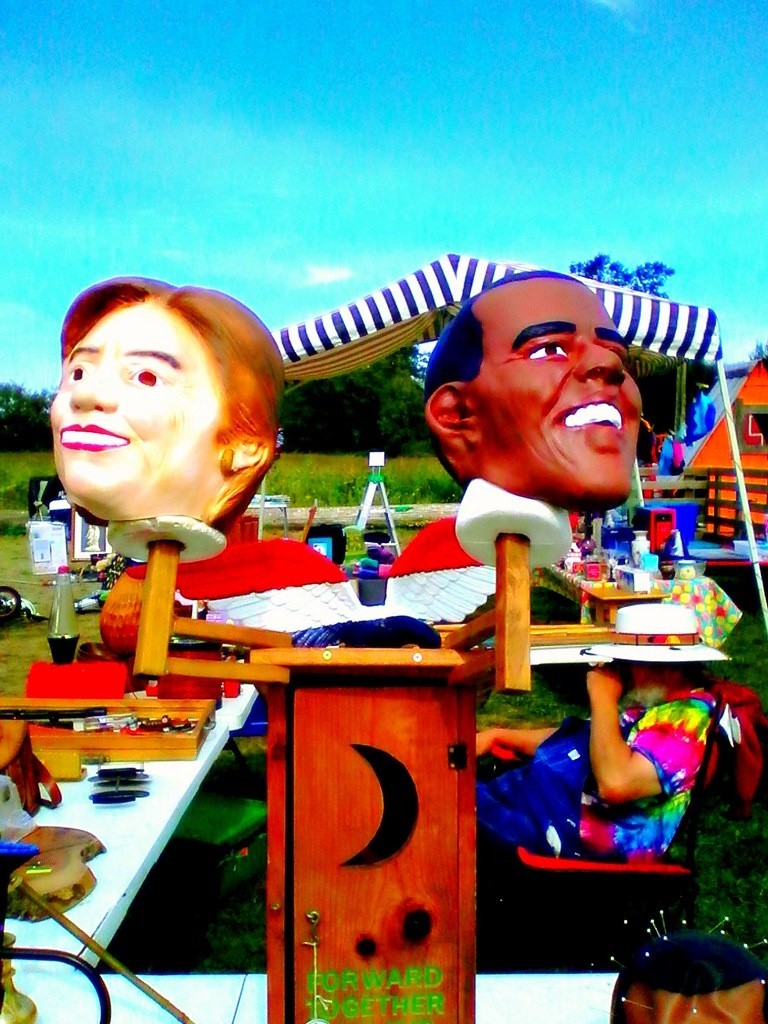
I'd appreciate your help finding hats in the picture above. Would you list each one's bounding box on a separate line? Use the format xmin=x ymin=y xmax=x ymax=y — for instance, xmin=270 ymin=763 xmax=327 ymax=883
xmin=589 ymin=602 xmax=731 ymax=663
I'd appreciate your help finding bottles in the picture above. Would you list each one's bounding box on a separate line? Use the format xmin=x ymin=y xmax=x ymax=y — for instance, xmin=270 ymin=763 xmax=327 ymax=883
xmin=661 ymin=561 xmax=675 ymax=580
xmin=579 ymin=525 xmax=595 ymax=556
xmin=679 ymin=560 xmax=695 ymax=580
xmin=631 ymin=530 xmax=650 ymax=567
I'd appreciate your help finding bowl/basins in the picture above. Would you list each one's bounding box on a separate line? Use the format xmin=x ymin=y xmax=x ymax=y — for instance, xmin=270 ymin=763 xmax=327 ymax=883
xmin=674 ymin=559 xmax=706 ymax=580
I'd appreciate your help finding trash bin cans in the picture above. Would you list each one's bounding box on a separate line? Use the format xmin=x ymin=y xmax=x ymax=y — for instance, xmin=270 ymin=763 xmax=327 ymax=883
xmin=307 ymin=524 xmax=346 ymax=565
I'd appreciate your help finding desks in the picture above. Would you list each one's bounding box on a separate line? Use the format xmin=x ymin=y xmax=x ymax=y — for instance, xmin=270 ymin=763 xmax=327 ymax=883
xmin=4 ymin=683 xmax=619 ymax=1023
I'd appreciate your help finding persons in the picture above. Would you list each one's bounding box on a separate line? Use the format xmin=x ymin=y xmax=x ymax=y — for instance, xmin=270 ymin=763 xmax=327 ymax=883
xmin=473 ymin=661 xmax=716 ymax=862
xmin=420 ymin=269 xmax=642 ymax=570
xmin=49 ymin=275 xmax=292 ymax=563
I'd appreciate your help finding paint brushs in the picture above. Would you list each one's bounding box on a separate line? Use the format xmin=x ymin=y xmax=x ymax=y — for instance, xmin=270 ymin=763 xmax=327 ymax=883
xmin=11 ymin=876 xmax=195 ymax=1024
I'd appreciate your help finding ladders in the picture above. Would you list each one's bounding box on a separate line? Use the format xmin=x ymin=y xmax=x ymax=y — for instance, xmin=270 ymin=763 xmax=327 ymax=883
xmin=356 ymin=452 xmax=400 ymax=558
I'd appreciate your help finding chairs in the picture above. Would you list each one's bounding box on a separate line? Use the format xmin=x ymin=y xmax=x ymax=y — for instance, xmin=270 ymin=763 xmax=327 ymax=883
xmin=515 ymin=676 xmax=768 ymax=932
